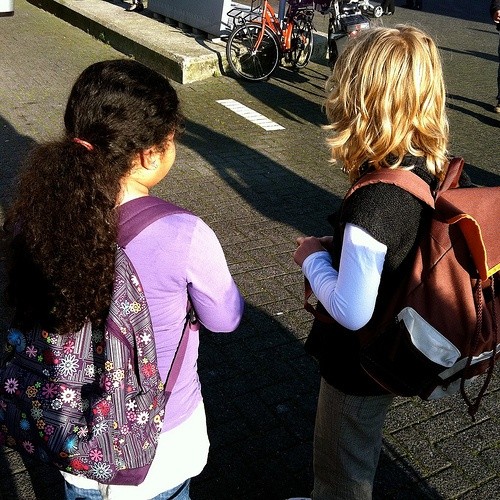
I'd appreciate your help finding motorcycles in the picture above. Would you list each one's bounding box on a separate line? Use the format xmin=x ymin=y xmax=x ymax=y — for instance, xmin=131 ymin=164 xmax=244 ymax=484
xmin=324 ymin=1 xmax=369 ymax=71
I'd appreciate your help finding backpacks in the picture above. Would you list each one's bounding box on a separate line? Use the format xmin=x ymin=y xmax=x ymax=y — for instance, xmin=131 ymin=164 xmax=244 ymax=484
xmin=0 ymin=197 xmax=200 ymax=486
xmin=304 ymin=157 xmax=500 ymax=396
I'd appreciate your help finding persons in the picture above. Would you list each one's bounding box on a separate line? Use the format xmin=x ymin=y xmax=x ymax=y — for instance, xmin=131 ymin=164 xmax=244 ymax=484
xmin=380 ymin=0 xmax=395 ymax=16
xmin=294 ymin=24 xmax=470 ymax=500
xmin=0 ymin=59 xmax=244 ymax=500
xmin=490 ymin=0 xmax=500 ymax=113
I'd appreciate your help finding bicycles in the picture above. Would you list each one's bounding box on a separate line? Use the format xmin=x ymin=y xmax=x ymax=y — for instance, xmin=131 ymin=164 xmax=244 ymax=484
xmin=225 ymin=1 xmax=315 ymax=83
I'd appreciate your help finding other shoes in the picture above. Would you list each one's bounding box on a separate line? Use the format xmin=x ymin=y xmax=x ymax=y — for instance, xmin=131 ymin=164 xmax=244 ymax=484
xmin=494 ymin=103 xmax=500 ymax=113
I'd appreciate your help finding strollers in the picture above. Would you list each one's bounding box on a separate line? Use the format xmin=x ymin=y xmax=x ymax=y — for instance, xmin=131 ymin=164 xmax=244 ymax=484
xmin=346 ymin=0 xmax=386 ymax=17
